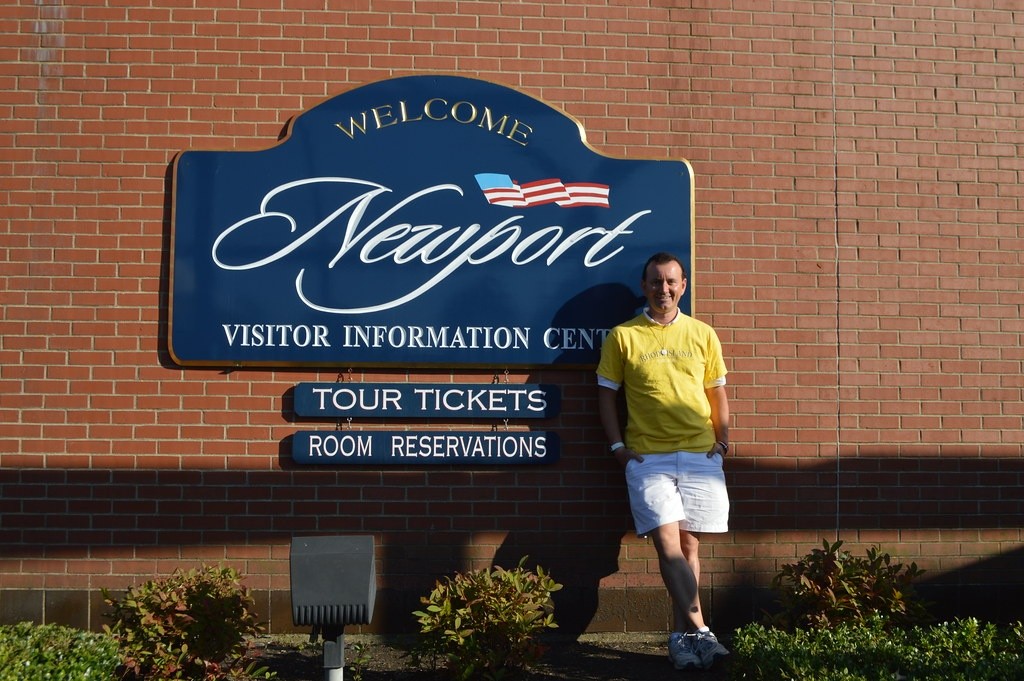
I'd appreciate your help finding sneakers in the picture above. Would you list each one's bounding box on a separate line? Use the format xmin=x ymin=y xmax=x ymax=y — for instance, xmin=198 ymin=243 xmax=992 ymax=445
xmin=691 ymin=631 xmax=732 ymax=671
xmin=668 ymin=631 xmax=703 ymax=670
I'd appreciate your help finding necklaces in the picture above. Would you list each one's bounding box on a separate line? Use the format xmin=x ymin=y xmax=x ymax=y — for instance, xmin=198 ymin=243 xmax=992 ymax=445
xmin=647 ymin=323 xmax=667 ymax=354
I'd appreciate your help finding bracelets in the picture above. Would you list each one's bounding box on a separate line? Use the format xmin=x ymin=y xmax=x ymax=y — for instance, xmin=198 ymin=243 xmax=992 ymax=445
xmin=717 ymin=441 xmax=729 ymax=452
xmin=610 ymin=442 xmax=625 ymax=455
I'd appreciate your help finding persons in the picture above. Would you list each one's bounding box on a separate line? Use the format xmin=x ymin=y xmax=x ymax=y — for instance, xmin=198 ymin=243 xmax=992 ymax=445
xmin=596 ymin=253 xmax=730 ymax=668
